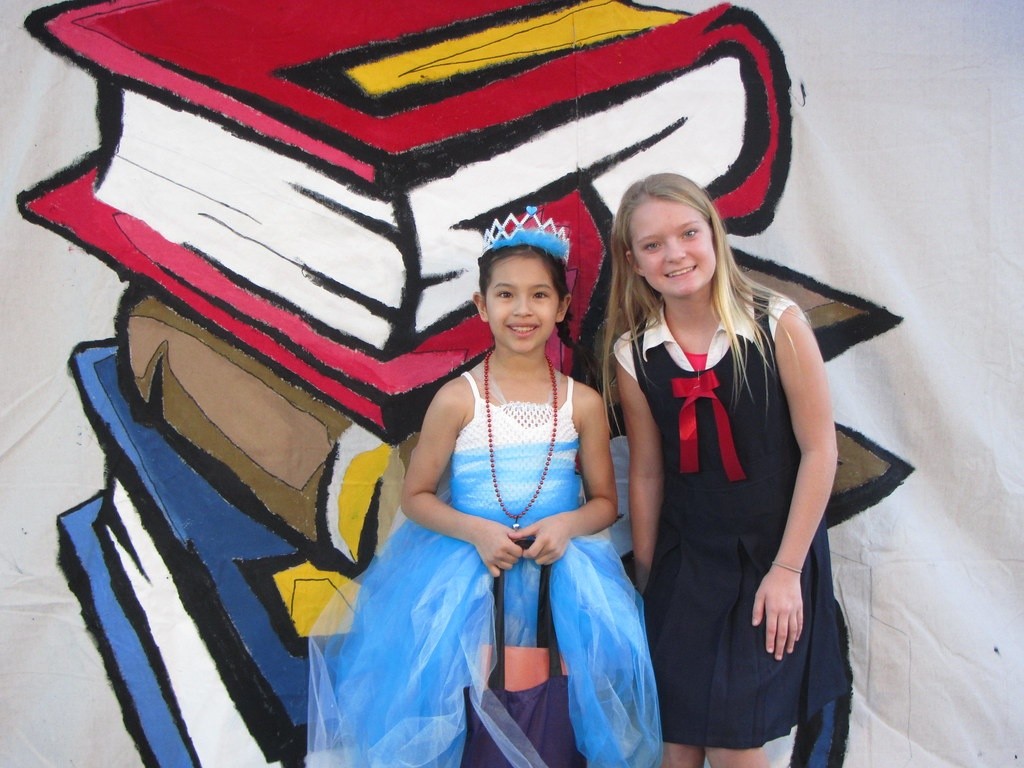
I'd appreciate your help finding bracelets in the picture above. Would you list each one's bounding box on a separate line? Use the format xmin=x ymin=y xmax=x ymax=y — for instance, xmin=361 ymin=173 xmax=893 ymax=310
xmin=771 ymin=561 xmax=803 ymax=574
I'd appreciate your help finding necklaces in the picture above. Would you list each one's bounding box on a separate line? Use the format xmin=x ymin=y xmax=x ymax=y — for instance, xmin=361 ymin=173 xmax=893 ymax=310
xmin=484 ymin=349 xmax=557 ymax=532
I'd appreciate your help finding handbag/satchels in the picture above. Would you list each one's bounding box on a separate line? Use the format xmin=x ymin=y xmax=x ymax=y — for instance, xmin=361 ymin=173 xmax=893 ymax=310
xmin=460 ymin=539 xmax=580 ymax=768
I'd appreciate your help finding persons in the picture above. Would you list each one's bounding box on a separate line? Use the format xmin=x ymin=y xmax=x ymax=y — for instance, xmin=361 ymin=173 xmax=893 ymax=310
xmin=305 ymin=206 xmax=665 ymax=768
xmin=601 ymin=173 xmax=849 ymax=768
